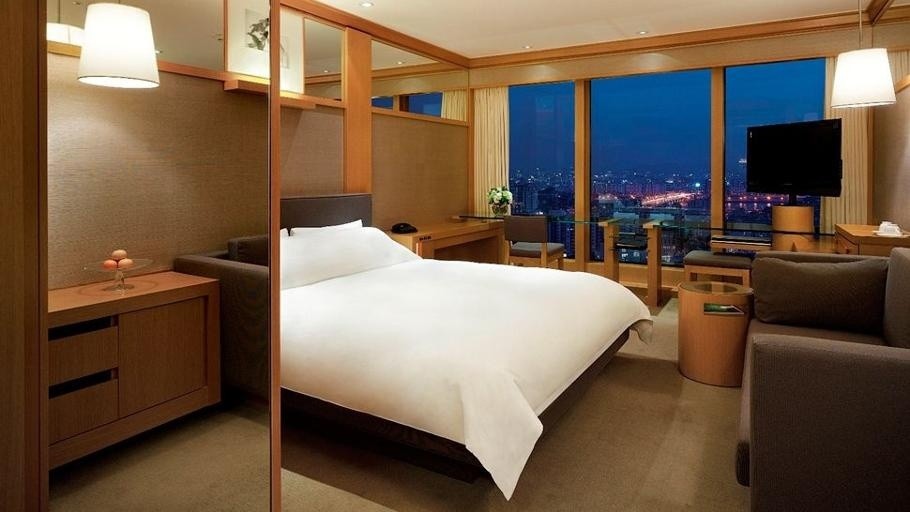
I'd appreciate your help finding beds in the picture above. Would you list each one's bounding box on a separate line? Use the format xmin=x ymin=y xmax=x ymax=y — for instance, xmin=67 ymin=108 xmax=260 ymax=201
xmin=281 ymin=188 xmax=656 ymax=502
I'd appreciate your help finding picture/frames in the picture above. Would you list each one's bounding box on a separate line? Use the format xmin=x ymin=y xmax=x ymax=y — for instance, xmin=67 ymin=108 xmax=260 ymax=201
xmin=224 ymin=0 xmax=306 ymax=95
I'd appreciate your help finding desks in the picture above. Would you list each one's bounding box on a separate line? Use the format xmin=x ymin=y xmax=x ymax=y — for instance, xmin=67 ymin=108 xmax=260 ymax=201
xmin=677 ymin=278 xmax=754 ymax=388
xmin=460 ymin=216 xmax=835 ymax=318
xmin=386 ymin=219 xmax=506 ymax=264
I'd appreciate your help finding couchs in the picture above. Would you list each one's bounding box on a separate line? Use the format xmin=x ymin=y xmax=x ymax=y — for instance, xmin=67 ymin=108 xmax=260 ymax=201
xmin=734 ymin=247 xmax=910 ymax=512
xmin=174 ymin=250 xmax=269 ymax=402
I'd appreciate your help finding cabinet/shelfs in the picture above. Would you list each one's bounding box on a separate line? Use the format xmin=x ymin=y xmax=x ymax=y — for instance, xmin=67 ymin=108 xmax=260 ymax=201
xmin=47 ymin=271 xmax=222 ymax=472
xmin=835 ymin=224 xmax=910 ymax=257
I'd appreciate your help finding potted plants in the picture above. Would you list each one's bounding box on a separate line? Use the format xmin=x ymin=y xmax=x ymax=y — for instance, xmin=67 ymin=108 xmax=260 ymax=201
xmin=485 ymin=185 xmax=513 ymax=215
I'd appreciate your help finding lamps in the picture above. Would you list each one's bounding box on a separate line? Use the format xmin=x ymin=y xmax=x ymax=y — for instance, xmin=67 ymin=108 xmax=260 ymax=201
xmin=76 ymin=0 xmax=160 ymax=89
xmin=829 ymin=0 xmax=897 ymax=108
xmin=46 ymin=0 xmax=84 ymax=47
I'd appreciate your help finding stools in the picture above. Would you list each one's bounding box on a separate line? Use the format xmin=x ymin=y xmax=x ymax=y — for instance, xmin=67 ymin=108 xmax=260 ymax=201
xmin=683 ymin=248 xmax=752 ymax=288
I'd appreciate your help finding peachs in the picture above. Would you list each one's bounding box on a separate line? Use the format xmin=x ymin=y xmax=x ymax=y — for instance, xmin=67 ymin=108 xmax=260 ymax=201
xmin=111 ymin=249 xmax=127 ymax=261
xmin=103 ymin=260 xmax=117 ymax=270
xmin=118 ymin=258 xmax=134 ymax=270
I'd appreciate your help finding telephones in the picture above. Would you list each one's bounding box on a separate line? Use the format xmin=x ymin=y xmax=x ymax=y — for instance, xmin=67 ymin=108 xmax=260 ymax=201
xmin=392 ymin=223 xmax=418 ymax=234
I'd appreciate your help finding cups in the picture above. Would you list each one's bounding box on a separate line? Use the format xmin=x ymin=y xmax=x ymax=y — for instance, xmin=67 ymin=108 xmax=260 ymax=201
xmin=878 ymin=220 xmax=902 ymax=235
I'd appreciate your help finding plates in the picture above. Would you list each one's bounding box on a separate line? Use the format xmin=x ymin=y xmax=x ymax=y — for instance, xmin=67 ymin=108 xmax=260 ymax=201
xmin=874 ymin=230 xmax=902 ymax=238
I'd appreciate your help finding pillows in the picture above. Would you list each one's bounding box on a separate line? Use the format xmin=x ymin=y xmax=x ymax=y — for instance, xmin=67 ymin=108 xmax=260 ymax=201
xmin=227 ymin=233 xmax=268 ymax=267
xmin=752 ymin=257 xmax=890 ymax=333
xmin=280 ymin=217 xmax=421 ymax=290
xmin=884 ymin=247 xmax=909 ymax=349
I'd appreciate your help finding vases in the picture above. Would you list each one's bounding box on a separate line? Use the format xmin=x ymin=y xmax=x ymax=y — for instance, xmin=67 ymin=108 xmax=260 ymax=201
xmin=491 ymin=202 xmax=509 ymax=218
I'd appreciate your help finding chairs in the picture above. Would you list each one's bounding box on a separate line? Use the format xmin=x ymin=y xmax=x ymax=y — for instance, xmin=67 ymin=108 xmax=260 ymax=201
xmin=504 ymin=215 xmax=565 ymax=270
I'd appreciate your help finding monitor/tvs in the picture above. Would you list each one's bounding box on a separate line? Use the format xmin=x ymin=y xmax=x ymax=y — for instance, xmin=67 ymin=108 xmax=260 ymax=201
xmin=746 ymin=118 xmax=842 ymax=207
